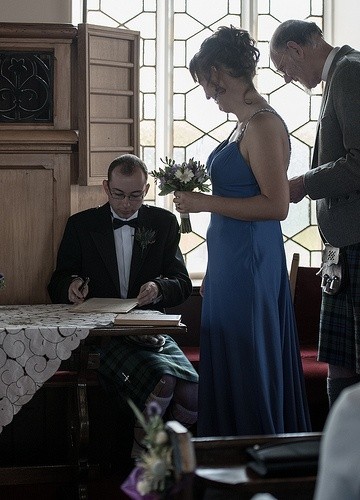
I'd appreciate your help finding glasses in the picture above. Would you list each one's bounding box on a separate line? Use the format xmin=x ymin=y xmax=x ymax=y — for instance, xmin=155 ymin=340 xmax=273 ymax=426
xmin=277 ymin=44 xmax=288 ymax=77
xmin=107 ymin=180 xmax=146 ymax=201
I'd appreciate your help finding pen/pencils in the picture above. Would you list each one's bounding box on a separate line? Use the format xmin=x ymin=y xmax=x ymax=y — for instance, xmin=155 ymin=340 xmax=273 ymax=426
xmin=74 ymin=276 xmax=91 ymax=299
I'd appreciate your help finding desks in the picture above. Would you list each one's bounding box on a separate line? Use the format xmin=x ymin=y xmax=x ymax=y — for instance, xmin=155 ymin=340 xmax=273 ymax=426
xmin=0 ymin=304 xmax=188 ymax=500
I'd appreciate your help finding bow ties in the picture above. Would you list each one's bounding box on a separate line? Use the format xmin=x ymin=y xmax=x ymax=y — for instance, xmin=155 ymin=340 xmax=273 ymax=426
xmin=111 ymin=212 xmax=140 ymax=230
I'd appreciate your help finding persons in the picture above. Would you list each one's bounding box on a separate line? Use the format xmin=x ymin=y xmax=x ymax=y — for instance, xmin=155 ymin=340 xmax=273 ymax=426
xmin=174 ymin=28 xmax=311 ymax=435
xmin=46 ymin=154 xmax=199 ymax=467
xmin=271 ymin=21 xmax=360 ymax=409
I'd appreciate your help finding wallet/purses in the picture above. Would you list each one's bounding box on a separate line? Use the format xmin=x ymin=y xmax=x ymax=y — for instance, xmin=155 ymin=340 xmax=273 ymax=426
xmin=245 ymin=438 xmax=322 ymax=477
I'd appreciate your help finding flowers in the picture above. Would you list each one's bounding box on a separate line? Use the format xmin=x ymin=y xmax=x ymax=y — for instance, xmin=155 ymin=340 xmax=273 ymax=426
xmin=120 ymin=392 xmax=175 ymax=500
xmin=0 ymin=272 xmax=8 ymax=289
xmin=147 ymin=156 xmax=213 ymax=234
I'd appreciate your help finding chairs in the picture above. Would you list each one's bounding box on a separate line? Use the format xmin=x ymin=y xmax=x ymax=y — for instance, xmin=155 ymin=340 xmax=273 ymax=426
xmin=290 ymin=253 xmax=328 ymax=432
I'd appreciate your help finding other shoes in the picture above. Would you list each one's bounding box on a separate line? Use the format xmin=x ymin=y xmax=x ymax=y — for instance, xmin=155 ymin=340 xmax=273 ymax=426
xmin=128 ymin=425 xmax=147 ymax=469
